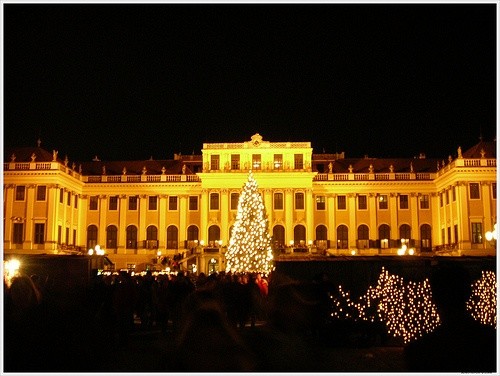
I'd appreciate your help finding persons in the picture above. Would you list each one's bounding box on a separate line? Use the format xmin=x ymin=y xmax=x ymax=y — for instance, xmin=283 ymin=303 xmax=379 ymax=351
xmin=4 ymin=245 xmax=496 ymax=373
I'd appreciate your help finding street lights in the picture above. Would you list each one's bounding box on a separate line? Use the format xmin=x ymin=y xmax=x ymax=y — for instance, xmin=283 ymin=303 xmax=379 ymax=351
xmin=485 ymin=224 xmax=497 ymax=257
xmin=87 ymin=245 xmax=104 ymax=269
